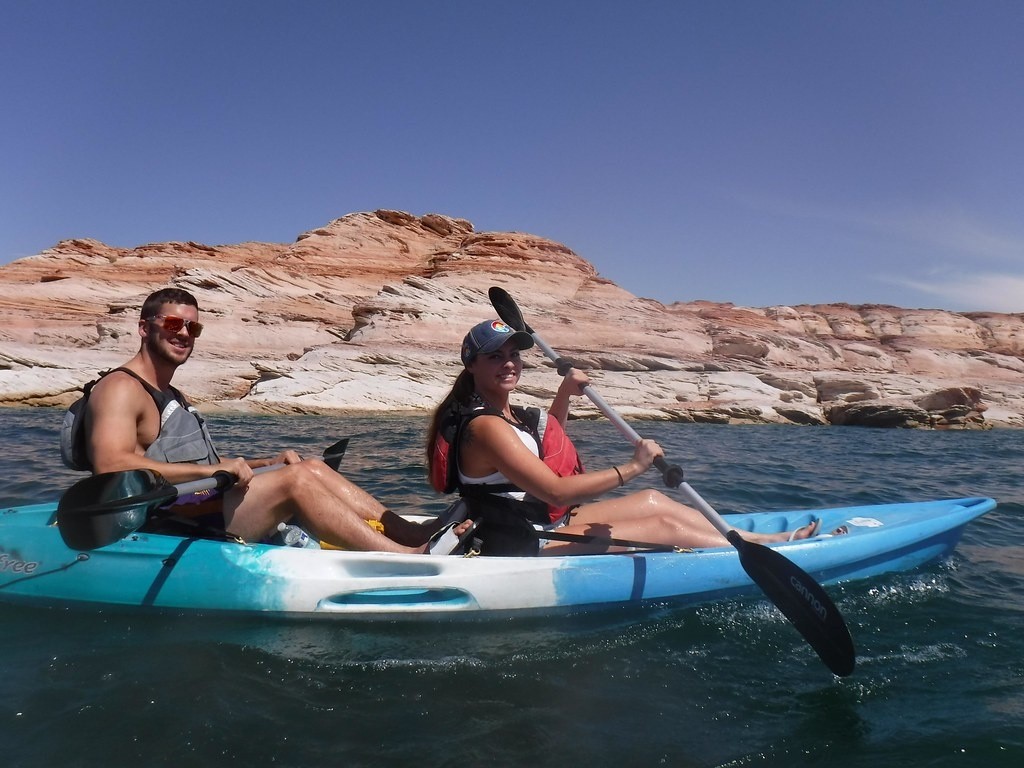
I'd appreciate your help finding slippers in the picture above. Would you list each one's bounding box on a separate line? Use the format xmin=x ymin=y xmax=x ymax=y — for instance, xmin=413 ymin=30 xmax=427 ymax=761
xmin=789 ymin=517 xmax=822 ymax=541
xmin=427 ymin=517 xmax=482 ymax=554
xmin=438 ymin=495 xmax=469 ymax=524
xmin=831 ymin=526 xmax=849 ymax=538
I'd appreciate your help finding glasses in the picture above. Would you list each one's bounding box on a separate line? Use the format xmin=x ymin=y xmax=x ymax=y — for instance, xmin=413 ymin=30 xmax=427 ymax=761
xmin=146 ymin=315 xmax=203 ymax=338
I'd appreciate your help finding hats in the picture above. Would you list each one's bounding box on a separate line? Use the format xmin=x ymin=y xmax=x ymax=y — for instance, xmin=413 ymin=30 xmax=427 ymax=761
xmin=461 ymin=318 xmax=534 ymax=370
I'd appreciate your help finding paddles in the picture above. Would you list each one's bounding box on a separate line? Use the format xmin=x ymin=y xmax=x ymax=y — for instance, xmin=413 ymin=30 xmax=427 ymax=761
xmin=56 ymin=436 xmax=349 ymax=553
xmin=488 ymin=284 xmax=857 ymax=679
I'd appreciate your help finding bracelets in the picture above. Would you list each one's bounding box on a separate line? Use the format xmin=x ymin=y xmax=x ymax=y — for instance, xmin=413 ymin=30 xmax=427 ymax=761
xmin=613 ymin=465 xmax=626 ymax=487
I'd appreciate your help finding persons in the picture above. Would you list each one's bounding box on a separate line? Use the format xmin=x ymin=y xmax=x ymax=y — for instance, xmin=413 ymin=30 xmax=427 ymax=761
xmin=84 ymin=288 xmax=484 ymax=555
xmin=426 ymin=320 xmax=850 ymax=557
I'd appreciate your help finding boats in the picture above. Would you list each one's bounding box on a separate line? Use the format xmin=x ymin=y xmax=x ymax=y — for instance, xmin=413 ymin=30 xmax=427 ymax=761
xmin=0 ymin=492 xmax=999 ymax=622
